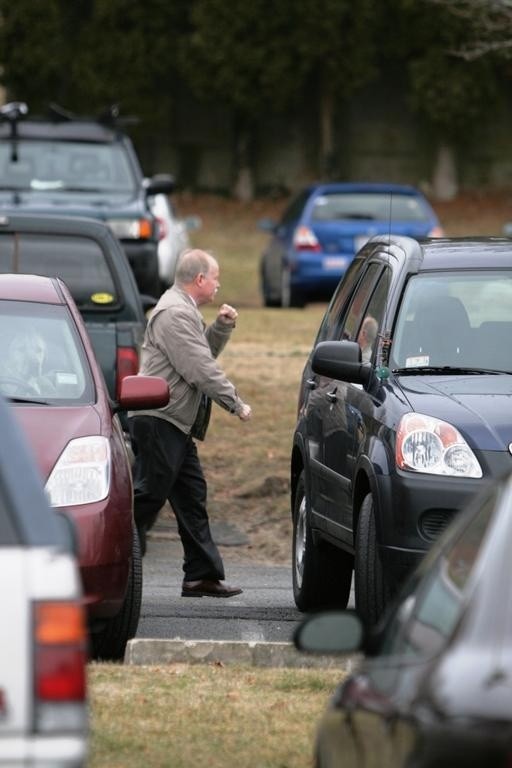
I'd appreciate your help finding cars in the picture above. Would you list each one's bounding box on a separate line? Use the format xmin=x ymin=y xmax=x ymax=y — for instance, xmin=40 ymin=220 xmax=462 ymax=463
xmin=257 ymin=184 xmax=443 ymax=310
xmin=3 ymin=273 xmax=173 ymax=662
xmin=290 ymin=467 xmax=510 ymax=765
xmin=141 ymin=177 xmax=204 ymax=286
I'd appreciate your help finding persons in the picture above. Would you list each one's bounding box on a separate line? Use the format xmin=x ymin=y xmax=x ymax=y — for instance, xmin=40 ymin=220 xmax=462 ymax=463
xmin=127 ymin=248 xmax=253 ymax=598
xmin=350 ymin=316 xmax=378 ymax=389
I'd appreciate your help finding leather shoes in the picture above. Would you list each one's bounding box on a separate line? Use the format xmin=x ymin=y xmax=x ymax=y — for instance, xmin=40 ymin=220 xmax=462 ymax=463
xmin=181 ymin=577 xmax=243 ymax=598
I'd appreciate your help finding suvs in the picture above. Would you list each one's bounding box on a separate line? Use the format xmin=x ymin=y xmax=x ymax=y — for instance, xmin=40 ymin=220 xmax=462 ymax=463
xmin=2 ymin=99 xmax=177 ymax=297
xmin=288 ymin=233 xmax=512 ymax=657
xmin=0 ymin=389 xmax=93 ymax=765
xmin=2 ymin=208 xmax=161 ymax=436
